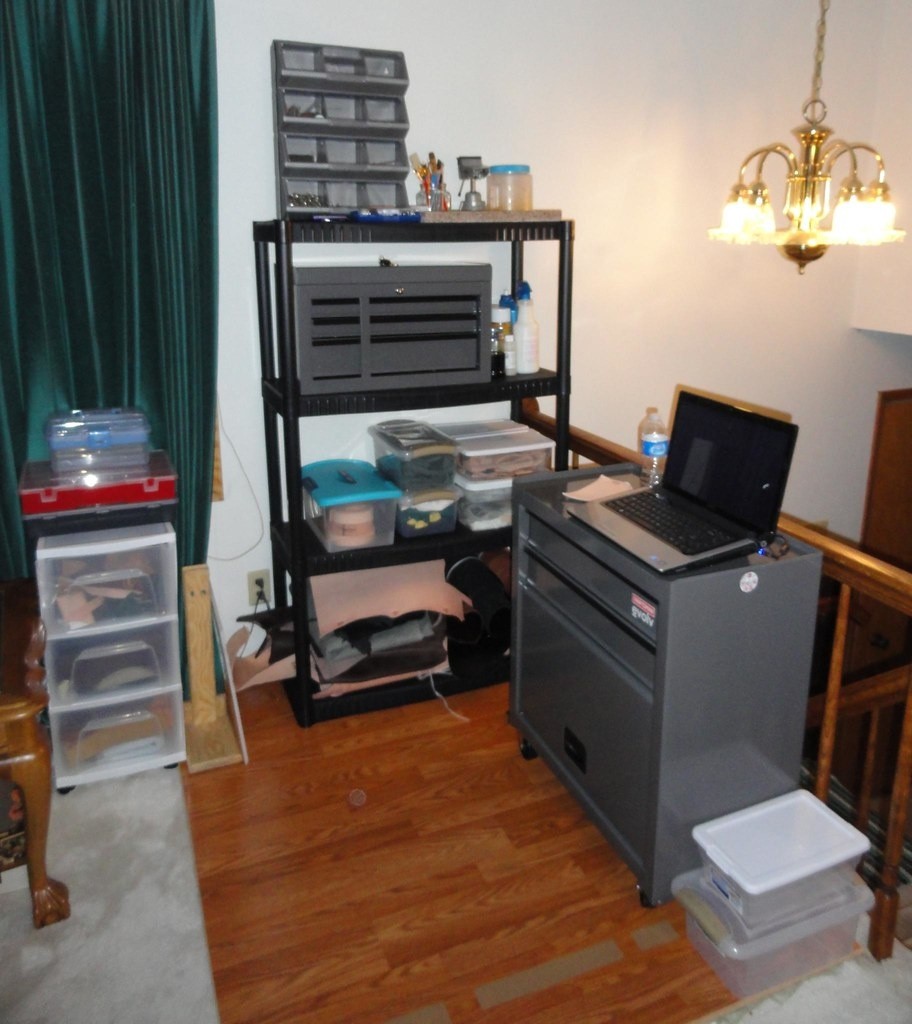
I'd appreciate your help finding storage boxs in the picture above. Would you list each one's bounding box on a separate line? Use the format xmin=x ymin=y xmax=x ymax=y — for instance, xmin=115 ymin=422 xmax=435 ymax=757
xmin=18 ymin=408 xmax=180 ymax=537
xmin=274 ymin=265 xmax=494 ymax=394
xmin=669 ymin=788 xmax=875 ymax=1000
xmin=301 ymin=419 xmax=554 ymax=554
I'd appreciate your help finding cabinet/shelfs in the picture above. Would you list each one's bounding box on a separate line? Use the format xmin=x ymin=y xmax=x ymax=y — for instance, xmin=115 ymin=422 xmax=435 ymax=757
xmin=509 ymin=461 xmax=824 ymax=909
xmin=35 ymin=522 xmax=188 ymax=795
xmin=273 ymin=42 xmax=411 ymax=220
xmin=254 ymin=218 xmax=577 ymax=730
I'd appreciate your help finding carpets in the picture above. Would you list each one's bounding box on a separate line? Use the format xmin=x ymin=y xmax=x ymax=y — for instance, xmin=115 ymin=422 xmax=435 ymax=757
xmin=688 ymin=955 xmax=912 ymax=1023
xmin=0 ymin=766 xmax=220 ymax=1024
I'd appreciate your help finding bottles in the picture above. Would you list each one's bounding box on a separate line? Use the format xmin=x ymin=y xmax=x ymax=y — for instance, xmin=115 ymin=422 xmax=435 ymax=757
xmin=639 ymin=411 xmax=669 ymax=489
xmin=490 ymin=290 xmax=518 ymax=380
xmin=416 ymin=183 xmax=451 ymax=213
xmin=485 ymin=164 xmax=534 ymax=213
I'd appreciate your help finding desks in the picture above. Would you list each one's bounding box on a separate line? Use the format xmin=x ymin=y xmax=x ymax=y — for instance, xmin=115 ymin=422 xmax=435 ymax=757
xmin=0 ymin=575 xmax=71 ymax=928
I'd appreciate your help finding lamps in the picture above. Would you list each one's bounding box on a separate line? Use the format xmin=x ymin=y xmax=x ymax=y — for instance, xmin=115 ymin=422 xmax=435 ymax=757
xmin=707 ymin=0 xmax=907 ymax=276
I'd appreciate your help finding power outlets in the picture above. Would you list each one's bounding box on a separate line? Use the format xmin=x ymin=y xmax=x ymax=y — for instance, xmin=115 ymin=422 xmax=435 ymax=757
xmin=248 ymin=569 xmax=269 ymax=605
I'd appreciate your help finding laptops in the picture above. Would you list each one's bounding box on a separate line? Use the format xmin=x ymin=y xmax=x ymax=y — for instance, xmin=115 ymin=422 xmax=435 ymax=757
xmin=567 ymin=390 xmax=799 ymax=576
